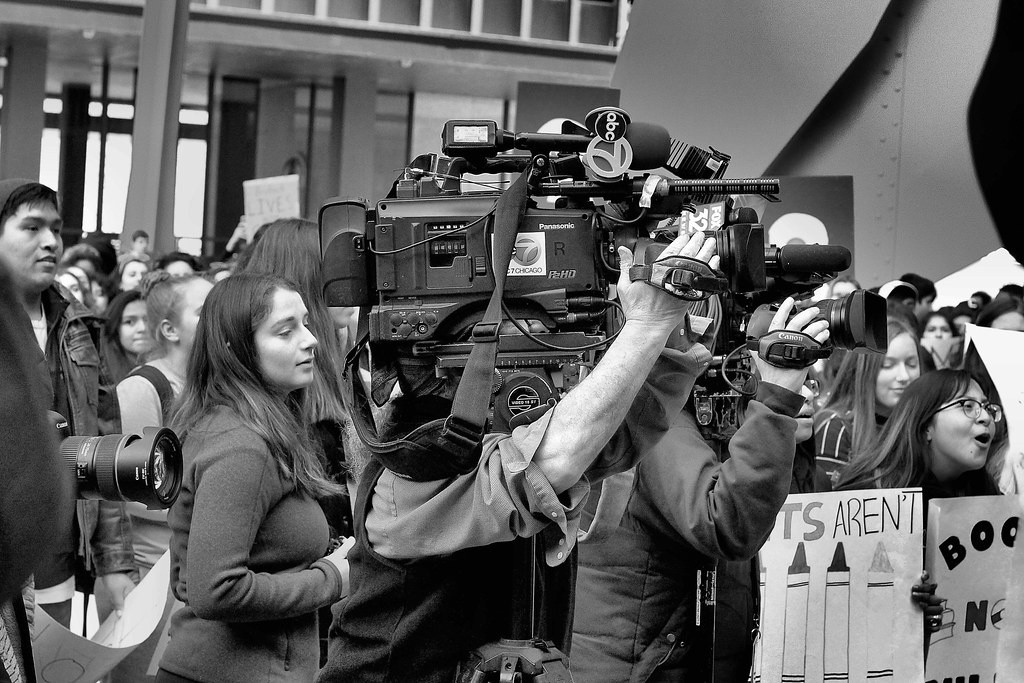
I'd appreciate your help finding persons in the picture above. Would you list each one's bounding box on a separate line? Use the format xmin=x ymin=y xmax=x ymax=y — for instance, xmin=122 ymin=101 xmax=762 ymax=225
xmin=1 ymin=176 xmax=138 ymax=683
xmin=313 ymin=197 xmax=730 ymax=682
xmin=563 ymin=249 xmax=831 ymax=682
xmin=111 ymin=265 xmax=215 ymax=582
xmin=761 ymin=213 xmax=1024 ymax=373
xmin=105 ymin=289 xmax=166 ymax=392
xmin=829 ymin=367 xmax=1013 ymax=682
xmin=959 ymin=295 xmax=1022 ymax=498
xmin=803 ymin=319 xmax=926 ymax=492
xmin=152 ymin=272 xmax=359 ymax=683
xmin=50 ymin=212 xmax=247 ymax=314
xmin=238 ymin=219 xmax=378 ymax=671
xmin=734 ymin=356 xmax=932 ymax=683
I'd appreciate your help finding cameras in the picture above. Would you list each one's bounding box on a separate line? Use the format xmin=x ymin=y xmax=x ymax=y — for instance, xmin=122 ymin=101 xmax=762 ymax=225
xmin=45 ymin=410 xmax=185 ymax=510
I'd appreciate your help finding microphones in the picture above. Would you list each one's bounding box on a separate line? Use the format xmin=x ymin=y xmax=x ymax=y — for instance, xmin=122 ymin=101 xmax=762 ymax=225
xmin=762 ymin=245 xmax=854 ymax=275
xmin=506 ymin=123 xmax=670 ymax=171
xmin=647 ymin=201 xmax=759 ymax=236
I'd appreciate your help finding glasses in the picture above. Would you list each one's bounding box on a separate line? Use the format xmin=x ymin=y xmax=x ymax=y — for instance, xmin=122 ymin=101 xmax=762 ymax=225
xmin=930 ymin=398 xmax=1002 ymax=422
